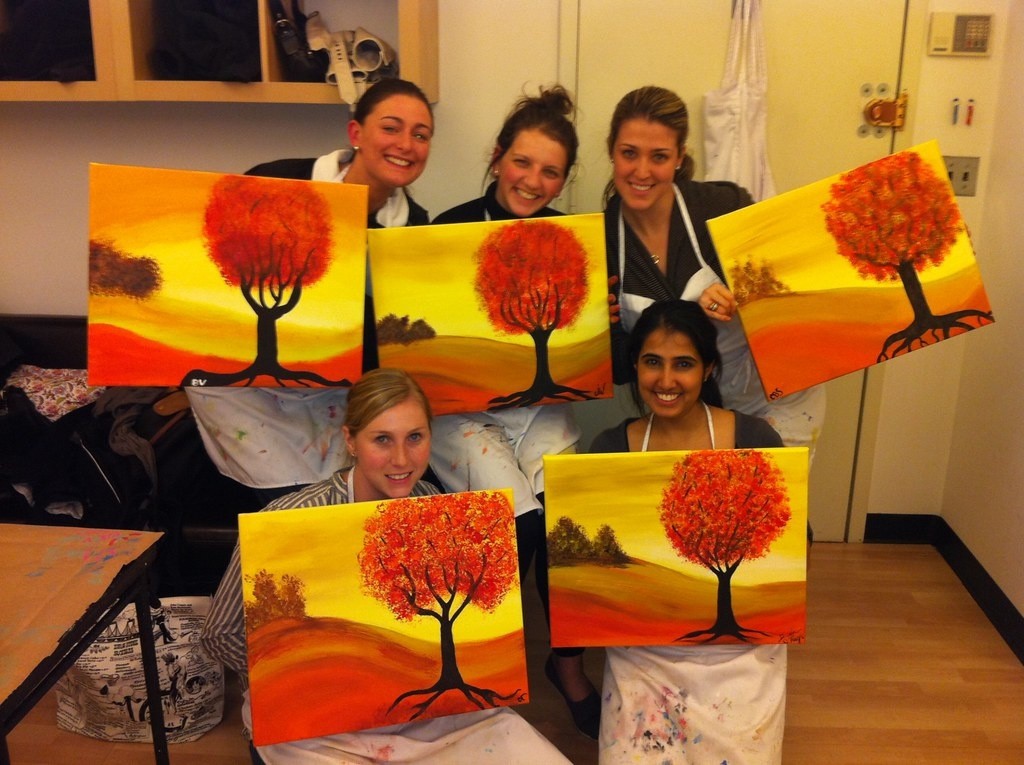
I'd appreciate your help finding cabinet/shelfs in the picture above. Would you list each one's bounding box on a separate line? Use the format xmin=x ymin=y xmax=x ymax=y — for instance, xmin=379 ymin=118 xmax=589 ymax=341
xmin=0 ymin=0 xmax=440 ymax=104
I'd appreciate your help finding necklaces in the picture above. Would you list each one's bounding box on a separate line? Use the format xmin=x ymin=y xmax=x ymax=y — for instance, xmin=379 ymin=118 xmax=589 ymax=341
xmin=649 ymin=253 xmax=665 ymax=266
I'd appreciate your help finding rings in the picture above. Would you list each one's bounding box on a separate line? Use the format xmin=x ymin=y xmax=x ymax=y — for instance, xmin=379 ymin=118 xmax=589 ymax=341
xmin=709 ymin=302 xmax=719 ymax=311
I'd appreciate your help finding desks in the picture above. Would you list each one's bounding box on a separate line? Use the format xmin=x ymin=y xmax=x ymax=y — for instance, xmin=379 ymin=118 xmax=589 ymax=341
xmin=0 ymin=521 xmax=171 ymax=765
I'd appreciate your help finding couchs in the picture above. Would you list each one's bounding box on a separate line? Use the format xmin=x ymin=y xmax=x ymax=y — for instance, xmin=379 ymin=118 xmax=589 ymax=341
xmin=0 ymin=313 xmax=264 ymax=550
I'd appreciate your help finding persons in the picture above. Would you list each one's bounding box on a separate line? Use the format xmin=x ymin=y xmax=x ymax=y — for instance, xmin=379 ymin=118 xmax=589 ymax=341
xmin=596 ymin=84 xmax=977 ymax=470
xmin=427 ymin=81 xmax=624 ymax=741
xmin=199 ymin=365 xmax=578 ymax=765
xmin=585 ymin=295 xmax=815 ymax=765
xmin=181 ymin=77 xmax=435 ymax=520
